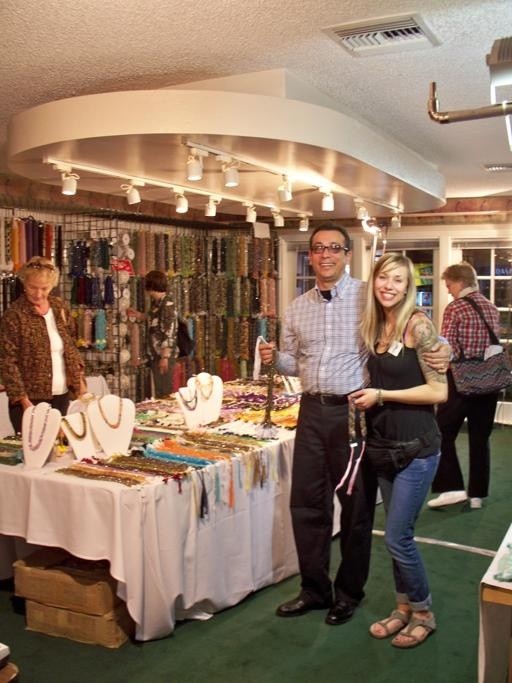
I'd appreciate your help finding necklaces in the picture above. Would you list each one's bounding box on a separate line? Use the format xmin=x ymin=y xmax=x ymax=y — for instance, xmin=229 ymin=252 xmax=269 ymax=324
xmin=196 ymin=374 xmax=213 ymax=399
xmin=254 ymin=336 xmax=276 ymax=429
xmin=128 ymin=277 xmax=155 ymax=312
xmin=2 ymin=216 xmax=62 ymax=275
xmin=221 ymin=374 xmax=301 ymax=440
xmin=186 ymin=316 xmax=280 ymax=360
xmin=62 ymin=413 xmax=87 ymax=440
xmin=127 ymin=366 xmax=154 ymax=401
xmin=98 ymin=396 xmax=122 ymax=430
xmin=170 ymin=360 xmax=270 ymax=387
xmin=79 ymin=394 xmax=95 ymax=403
xmin=128 ymin=321 xmax=148 ymax=368
xmin=167 ymin=275 xmax=277 ymax=314
xmin=56 ymin=430 xmax=269 ymax=520
xmin=0 ymin=435 xmax=23 ymax=466
xmin=29 ymin=404 xmax=53 ymax=451
xmin=133 ymin=399 xmax=185 ymax=430
xmin=177 ymin=389 xmax=197 ymax=411
xmin=133 ymin=230 xmax=278 ymax=277
xmin=70 ymin=238 xmax=114 ymax=349
xmin=334 ymin=398 xmax=367 ymax=495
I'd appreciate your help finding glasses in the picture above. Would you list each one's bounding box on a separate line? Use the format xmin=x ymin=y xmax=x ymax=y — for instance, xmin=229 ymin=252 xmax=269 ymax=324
xmin=309 ymin=243 xmax=350 ymax=254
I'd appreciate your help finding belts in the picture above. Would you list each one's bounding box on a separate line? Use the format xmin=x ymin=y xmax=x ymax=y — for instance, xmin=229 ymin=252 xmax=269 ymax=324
xmin=306 ymin=391 xmax=353 ymax=407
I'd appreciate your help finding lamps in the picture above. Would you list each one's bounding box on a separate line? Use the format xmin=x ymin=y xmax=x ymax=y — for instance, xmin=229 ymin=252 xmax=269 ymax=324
xmin=126 ymin=189 xmax=141 ymax=205
xmin=279 ymin=185 xmax=293 ymax=202
xmin=188 ymin=160 xmax=202 ymax=181
xmin=204 ymin=203 xmax=216 ymax=216
xmin=298 ymin=219 xmax=308 ymax=232
xmin=321 ymin=193 xmax=334 ymax=212
xmin=245 ymin=208 xmax=257 ymax=223
xmin=391 ymin=217 xmax=401 ymax=229
xmin=223 ymin=168 xmax=239 ymax=188
xmin=273 ymin=215 xmax=285 ymax=227
xmin=176 ymin=198 xmax=189 ymax=214
xmin=60 ymin=177 xmax=77 ymax=196
xmin=357 ymin=208 xmax=365 ymax=220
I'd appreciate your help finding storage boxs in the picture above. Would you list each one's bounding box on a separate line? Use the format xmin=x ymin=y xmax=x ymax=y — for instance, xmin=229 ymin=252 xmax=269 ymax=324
xmin=12 ymin=550 xmax=123 ymax=615
xmin=25 ymin=600 xmax=139 ymax=648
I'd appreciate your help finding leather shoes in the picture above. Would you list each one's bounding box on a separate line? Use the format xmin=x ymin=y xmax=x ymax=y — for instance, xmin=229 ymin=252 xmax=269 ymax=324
xmin=277 ymin=592 xmax=312 ymax=617
xmin=325 ymin=600 xmax=356 ymax=624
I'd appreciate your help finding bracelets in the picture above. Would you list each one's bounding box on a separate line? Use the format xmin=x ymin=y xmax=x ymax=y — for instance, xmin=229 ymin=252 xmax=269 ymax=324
xmin=376 ymin=390 xmax=383 ymax=407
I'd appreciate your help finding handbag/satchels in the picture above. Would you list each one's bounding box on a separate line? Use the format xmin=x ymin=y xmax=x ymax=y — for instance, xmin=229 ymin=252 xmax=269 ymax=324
xmin=362 ymin=427 xmax=439 ymax=471
xmin=451 ymin=296 xmax=512 ymax=395
xmin=177 ymin=317 xmax=196 ymax=360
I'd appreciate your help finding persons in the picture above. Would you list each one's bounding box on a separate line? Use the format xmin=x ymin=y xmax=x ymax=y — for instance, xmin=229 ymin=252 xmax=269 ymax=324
xmin=426 ymin=263 xmax=500 ymax=509
xmin=260 ymin=223 xmax=452 ymax=626
xmin=126 ymin=270 xmax=177 ymax=400
xmin=347 ymin=253 xmax=448 ymax=647
xmin=0 ymin=256 xmax=85 ymax=435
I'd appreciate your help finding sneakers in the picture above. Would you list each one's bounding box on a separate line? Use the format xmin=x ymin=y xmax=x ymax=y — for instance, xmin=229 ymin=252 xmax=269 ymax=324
xmin=427 ymin=491 xmax=466 ymax=508
xmin=470 ymin=498 xmax=480 ymax=507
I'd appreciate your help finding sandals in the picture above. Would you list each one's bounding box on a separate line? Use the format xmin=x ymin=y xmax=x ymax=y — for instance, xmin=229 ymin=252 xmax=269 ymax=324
xmin=391 ymin=610 xmax=436 ymax=648
xmin=368 ymin=608 xmax=411 ymax=638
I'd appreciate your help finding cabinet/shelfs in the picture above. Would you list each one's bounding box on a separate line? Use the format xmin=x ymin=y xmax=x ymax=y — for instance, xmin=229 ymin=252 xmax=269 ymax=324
xmin=0 ymin=377 xmax=301 ymax=646
xmin=483 ymin=518 xmax=512 ymax=683
